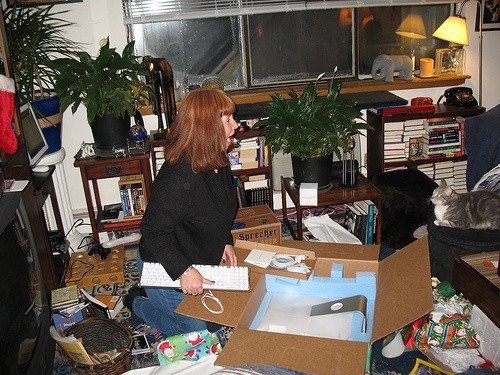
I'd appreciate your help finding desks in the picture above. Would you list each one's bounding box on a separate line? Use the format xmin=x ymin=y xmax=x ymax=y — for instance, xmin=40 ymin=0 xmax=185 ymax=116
xmin=280 ymin=172 xmax=382 ymax=242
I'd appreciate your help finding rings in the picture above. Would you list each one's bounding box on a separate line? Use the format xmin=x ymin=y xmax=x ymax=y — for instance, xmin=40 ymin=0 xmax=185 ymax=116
xmin=193 ymin=293 xmax=197 ymax=295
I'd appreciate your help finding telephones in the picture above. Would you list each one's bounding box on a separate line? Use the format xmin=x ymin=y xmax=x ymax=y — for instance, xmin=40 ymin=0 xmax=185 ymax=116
xmin=445 ymin=87 xmax=478 ymax=109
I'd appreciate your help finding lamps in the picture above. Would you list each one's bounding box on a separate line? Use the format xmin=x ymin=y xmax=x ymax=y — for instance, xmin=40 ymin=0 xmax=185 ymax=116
xmin=396 ymin=5 xmax=427 ymax=39
xmin=432 ymin=0 xmax=482 ymax=107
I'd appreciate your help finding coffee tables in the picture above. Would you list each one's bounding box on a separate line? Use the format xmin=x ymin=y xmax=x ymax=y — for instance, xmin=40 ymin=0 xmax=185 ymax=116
xmin=454 ymin=251 xmax=500 ymax=329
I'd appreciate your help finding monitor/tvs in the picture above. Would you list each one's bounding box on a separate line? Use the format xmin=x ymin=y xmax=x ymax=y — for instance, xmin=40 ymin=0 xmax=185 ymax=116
xmin=18 ymin=102 xmax=48 ymax=170
xmin=0 ymin=190 xmax=56 ymax=375
xmin=256 ymin=291 xmax=367 ymax=341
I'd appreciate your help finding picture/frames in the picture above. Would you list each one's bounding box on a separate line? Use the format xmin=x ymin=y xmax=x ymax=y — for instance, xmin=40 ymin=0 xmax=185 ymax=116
xmin=480 ymin=0 xmax=500 ymax=31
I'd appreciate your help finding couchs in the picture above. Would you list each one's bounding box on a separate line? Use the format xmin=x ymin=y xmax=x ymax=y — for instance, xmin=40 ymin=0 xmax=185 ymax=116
xmin=374 ymin=104 xmax=500 ymax=288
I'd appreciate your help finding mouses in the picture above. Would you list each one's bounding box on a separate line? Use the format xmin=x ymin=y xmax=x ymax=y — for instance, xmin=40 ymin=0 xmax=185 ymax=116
xmin=32 ymin=166 xmax=49 ymax=173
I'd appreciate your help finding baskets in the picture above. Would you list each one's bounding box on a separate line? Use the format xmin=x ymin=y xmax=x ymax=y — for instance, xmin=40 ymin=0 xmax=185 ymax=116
xmin=58 ymin=317 xmax=134 ymax=375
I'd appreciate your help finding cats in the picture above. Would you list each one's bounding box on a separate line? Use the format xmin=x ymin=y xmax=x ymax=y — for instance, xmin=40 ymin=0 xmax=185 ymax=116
xmin=426 ymin=177 xmax=500 ymax=230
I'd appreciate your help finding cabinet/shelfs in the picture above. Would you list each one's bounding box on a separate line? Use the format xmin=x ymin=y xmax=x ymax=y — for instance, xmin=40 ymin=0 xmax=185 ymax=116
xmin=368 ymin=105 xmax=486 ymax=198
xmin=73 ymin=145 xmax=151 ymax=254
xmin=0 ymin=0 xmax=70 ymax=295
xmin=150 ymin=125 xmax=272 ymax=210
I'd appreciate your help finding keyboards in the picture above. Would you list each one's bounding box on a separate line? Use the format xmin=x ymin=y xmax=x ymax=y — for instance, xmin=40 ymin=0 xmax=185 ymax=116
xmin=139 ymin=262 xmax=251 ymax=291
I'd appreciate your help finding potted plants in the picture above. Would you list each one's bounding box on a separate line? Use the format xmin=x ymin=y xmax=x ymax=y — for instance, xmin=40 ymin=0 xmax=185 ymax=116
xmin=0 ymin=0 xmax=90 ymax=164
xmin=49 ymin=36 xmax=154 ymax=153
xmin=252 ymin=65 xmax=376 ymax=189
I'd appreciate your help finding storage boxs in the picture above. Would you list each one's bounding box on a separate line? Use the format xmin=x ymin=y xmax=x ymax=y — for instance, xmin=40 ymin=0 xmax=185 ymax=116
xmin=65 ymin=203 xmax=435 ymax=375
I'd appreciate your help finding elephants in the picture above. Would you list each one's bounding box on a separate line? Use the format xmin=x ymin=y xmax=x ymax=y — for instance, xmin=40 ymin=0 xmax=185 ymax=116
xmin=370 ymin=53 xmax=414 ymax=83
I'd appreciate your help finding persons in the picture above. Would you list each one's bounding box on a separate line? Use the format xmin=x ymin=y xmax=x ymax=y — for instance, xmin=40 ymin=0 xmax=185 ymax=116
xmin=123 ymin=88 xmax=239 ymax=339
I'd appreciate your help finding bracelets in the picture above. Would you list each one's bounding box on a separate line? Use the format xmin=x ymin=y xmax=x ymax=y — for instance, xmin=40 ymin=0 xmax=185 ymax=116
xmin=182 ymin=266 xmax=193 ymax=274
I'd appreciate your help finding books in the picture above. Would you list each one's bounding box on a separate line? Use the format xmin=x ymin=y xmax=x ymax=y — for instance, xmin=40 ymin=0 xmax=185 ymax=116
xmin=384 ymin=116 xmax=468 ymax=195
xmin=244 ymin=174 xmax=270 ymax=206
xmin=303 ymin=232 xmax=318 ymax=242
xmin=344 ymin=200 xmax=377 ymax=245
xmin=154 ymin=146 xmax=165 ymax=176
xmin=49 ymin=285 xmax=123 ymax=365
xmin=229 ymin=136 xmax=268 ymax=170
xmin=120 ymin=183 xmax=145 ymax=217
